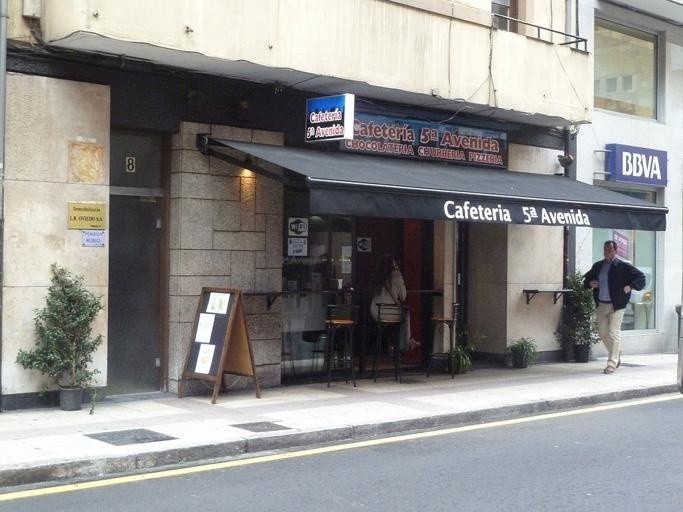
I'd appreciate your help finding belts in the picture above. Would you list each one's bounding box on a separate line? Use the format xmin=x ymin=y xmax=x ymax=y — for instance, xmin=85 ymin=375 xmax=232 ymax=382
xmin=599 ymin=301 xmax=613 ymax=303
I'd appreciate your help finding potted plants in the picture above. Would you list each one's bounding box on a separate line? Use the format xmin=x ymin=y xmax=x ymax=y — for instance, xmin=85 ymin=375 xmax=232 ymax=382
xmin=553 ymin=270 xmax=602 ymax=362
xmin=446 ymin=345 xmax=471 ymax=374
xmin=503 ymin=336 xmax=538 ymax=368
xmin=15 ymin=261 xmax=110 ymax=417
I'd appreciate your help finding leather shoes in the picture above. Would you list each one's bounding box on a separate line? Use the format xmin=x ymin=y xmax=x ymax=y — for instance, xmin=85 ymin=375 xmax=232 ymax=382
xmin=604 ymin=366 xmax=615 ymax=374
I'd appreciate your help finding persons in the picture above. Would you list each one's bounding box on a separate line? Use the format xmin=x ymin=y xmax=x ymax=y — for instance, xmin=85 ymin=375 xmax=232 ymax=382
xmin=367 ymin=257 xmax=422 ymax=354
xmin=581 ymin=240 xmax=645 ymax=375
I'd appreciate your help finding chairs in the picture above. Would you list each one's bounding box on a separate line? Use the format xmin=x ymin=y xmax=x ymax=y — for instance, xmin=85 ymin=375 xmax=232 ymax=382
xmin=321 ymin=302 xmax=407 ymax=388
xmin=425 ymin=303 xmax=460 ymax=380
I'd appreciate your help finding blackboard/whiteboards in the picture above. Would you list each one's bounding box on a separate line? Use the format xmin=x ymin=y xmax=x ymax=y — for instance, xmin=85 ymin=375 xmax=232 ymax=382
xmin=182 ymin=287 xmax=241 ymax=382
